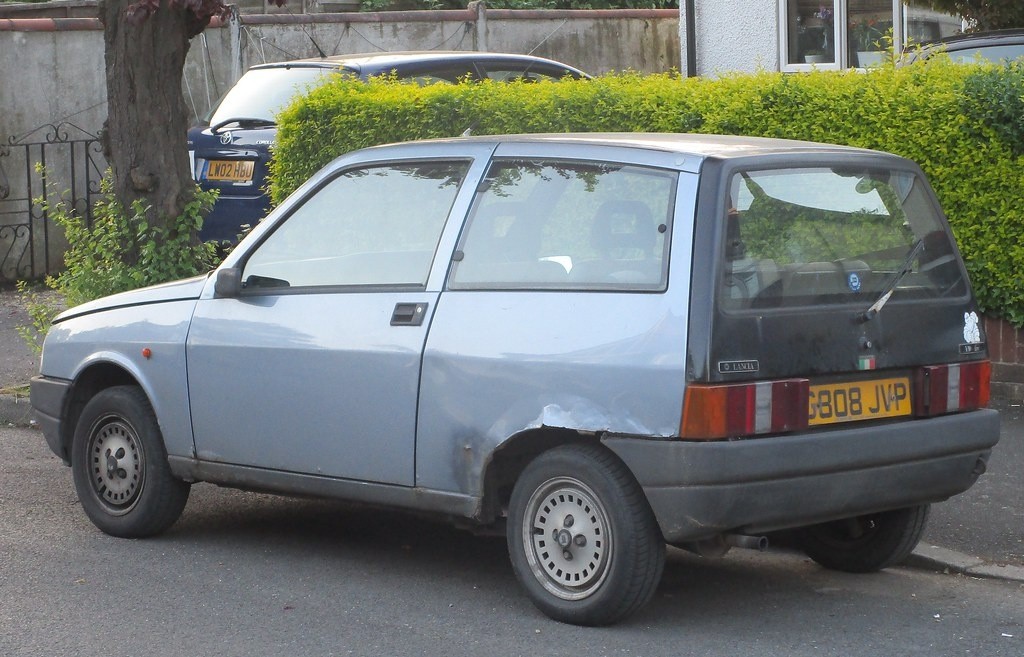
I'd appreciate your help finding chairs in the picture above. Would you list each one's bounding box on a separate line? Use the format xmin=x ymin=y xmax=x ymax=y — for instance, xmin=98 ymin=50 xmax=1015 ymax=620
xmin=564 ymin=197 xmax=662 ymax=288
xmin=456 ymin=201 xmax=568 ymax=286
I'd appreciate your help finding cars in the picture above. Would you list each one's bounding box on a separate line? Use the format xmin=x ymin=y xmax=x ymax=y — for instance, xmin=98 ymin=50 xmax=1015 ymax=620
xmin=893 ymin=31 xmax=1023 ymax=111
xmin=194 ymin=52 xmax=605 ymax=244
xmin=31 ymin=134 xmax=1000 ymax=626
